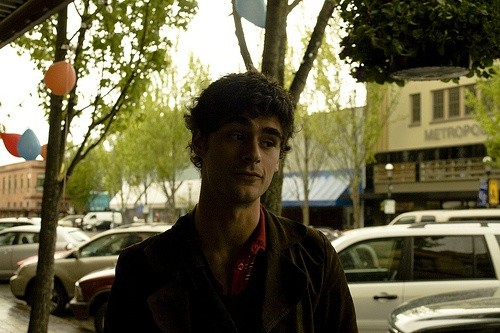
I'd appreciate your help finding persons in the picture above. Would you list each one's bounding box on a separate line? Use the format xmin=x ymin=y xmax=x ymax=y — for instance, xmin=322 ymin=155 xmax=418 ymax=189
xmin=105 ymin=70 xmax=360 ymax=333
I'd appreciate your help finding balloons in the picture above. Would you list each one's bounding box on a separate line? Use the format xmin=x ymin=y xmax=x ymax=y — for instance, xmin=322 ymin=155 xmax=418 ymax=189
xmin=0 ymin=127 xmax=48 ymax=162
xmin=45 ymin=61 xmax=77 ymax=96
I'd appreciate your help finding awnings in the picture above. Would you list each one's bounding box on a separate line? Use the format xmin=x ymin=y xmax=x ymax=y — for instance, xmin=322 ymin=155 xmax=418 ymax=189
xmin=110 ymin=178 xmax=201 ymax=209
xmin=283 ymin=173 xmax=362 ymax=207
xmin=88 ymin=193 xmax=110 ymax=211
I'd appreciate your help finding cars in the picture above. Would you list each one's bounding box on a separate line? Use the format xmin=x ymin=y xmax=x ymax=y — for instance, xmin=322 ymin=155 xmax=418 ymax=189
xmin=0 ymin=225 xmax=92 ymax=282
xmin=0 ymin=217 xmax=41 ymax=232
xmin=386 ymin=208 xmax=500 ymax=225
xmin=57 ymin=211 xmax=123 ymax=231
xmin=328 ymin=220 xmax=500 ymax=333
xmin=66 ymin=269 xmax=116 ymax=333
xmin=8 ymin=222 xmax=172 ymax=314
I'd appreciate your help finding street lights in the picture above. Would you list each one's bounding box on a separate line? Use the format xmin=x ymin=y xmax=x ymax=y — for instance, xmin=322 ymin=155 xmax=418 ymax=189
xmin=483 ymin=156 xmax=492 ymax=208
xmin=384 ymin=163 xmax=393 ymax=222
